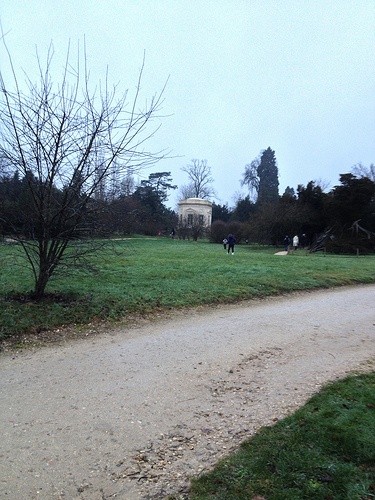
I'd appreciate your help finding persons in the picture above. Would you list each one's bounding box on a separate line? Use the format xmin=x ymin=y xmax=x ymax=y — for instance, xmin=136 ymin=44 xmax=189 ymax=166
xmin=284 ymin=235 xmax=291 ymax=251
xmin=292 ymin=234 xmax=300 ymax=250
xmin=222 ymin=237 xmax=229 ymax=249
xmin=227 ymin=233 xmax=236 ymax=255
